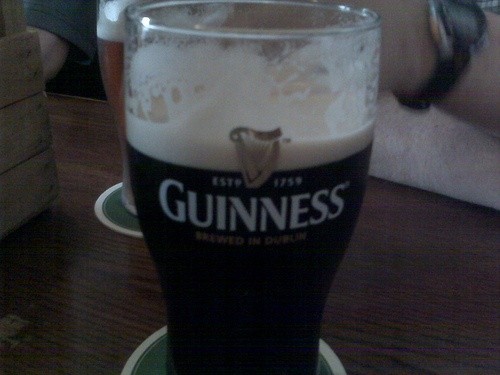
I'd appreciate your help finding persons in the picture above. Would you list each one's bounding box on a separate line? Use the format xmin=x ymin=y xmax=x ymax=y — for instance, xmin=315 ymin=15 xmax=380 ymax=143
xmin=23 ymin=0 xmax=107 ymax=100
xmin=214 ymin=0 xmax=500 ymax=137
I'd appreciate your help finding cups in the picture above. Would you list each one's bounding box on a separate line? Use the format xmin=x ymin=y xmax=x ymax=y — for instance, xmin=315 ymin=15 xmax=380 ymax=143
xmin=95 ymin=0 xmax=140 ymax=215
xmin=119 ymin=0 xmax=386 ymax=374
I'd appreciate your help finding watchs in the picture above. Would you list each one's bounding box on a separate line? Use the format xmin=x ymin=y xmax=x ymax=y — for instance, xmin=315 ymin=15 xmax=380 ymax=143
xmin=391 ymin=0 xmax=488 ymax=112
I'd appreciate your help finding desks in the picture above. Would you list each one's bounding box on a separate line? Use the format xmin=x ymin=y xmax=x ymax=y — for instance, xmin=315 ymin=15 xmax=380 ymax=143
xmin=1 ymin=93 xmax=500 ymax=375
